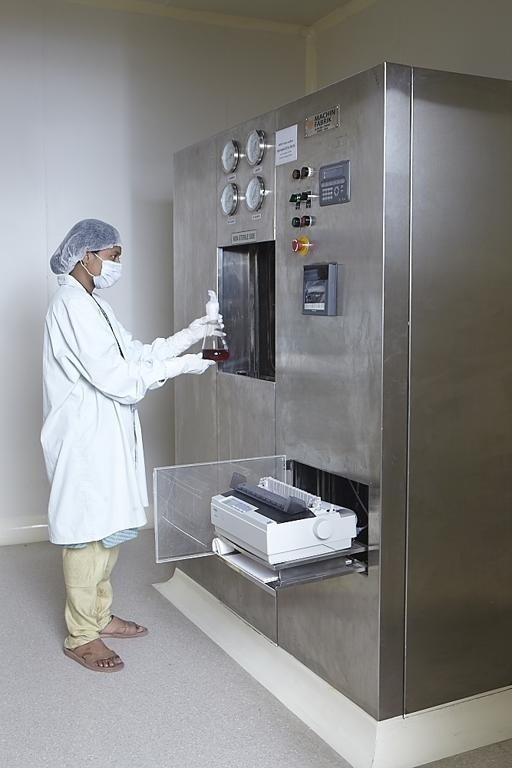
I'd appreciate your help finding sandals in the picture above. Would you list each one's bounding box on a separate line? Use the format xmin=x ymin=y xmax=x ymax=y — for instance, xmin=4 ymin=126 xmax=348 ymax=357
xmin=63 ymin=615 xmax=149 ymax=673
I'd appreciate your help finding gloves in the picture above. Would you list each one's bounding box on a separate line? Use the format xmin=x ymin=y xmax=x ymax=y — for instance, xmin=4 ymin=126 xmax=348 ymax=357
xmin=152 ymin=314 xmax=227 ymax=359
xmin=139 ymin=352 xmax=216 ymax=391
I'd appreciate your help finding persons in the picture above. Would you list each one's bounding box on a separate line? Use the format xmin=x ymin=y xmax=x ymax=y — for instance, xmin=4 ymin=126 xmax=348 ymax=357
xmin=40 ymin=218 xmax=226 ymax=672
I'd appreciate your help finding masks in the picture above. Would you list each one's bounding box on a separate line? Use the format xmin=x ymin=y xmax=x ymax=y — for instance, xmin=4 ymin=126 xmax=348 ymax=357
xmin=80 ymin=253 xmax=122 ymax=289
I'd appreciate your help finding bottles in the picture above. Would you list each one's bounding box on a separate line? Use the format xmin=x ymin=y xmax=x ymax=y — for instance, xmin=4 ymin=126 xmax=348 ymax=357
xmin=203 ymin=302 xmax=231 ymax=362
